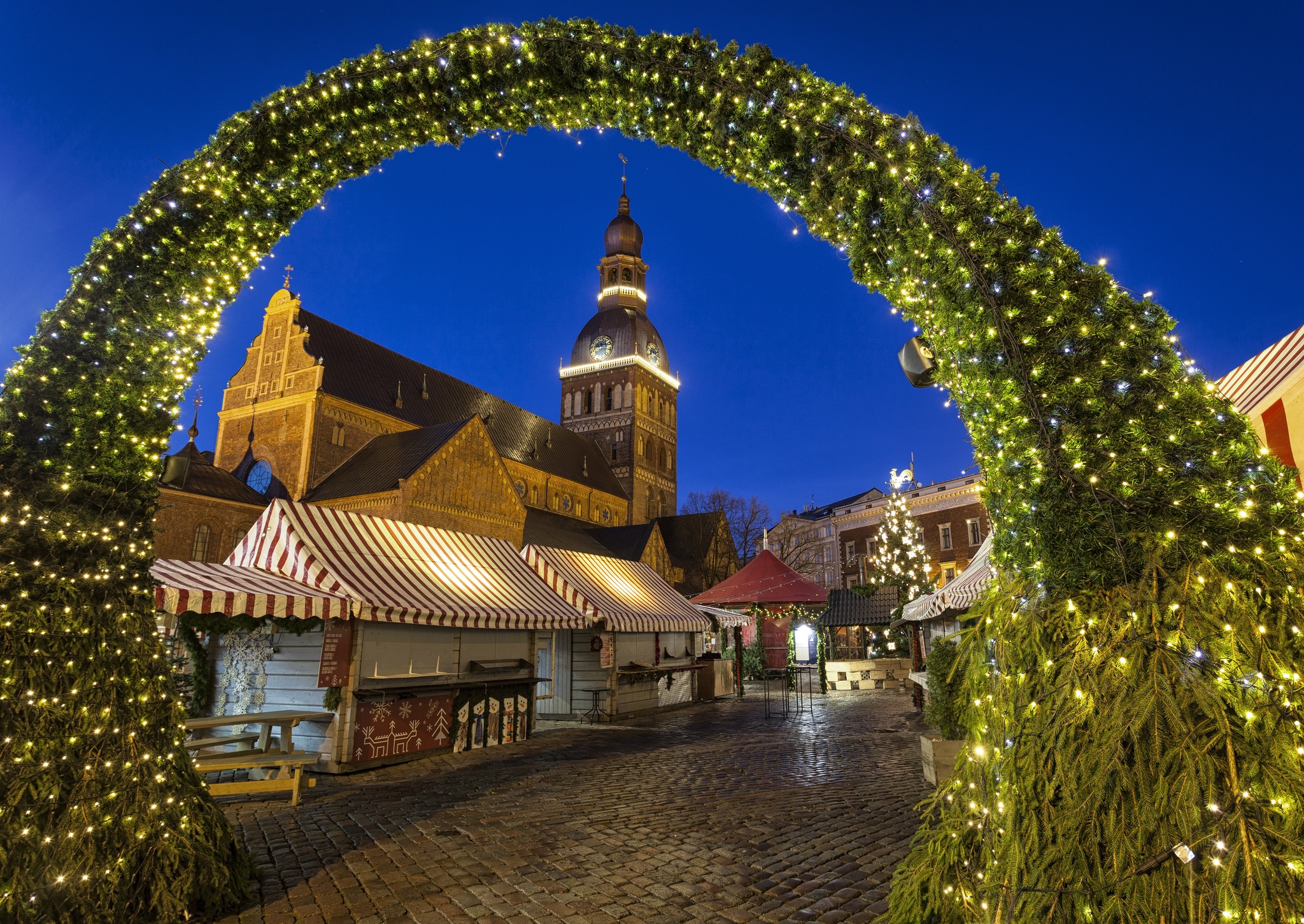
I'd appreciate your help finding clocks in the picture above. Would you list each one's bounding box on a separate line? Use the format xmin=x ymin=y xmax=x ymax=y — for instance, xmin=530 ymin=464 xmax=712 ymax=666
xmin=590 ymin=336 xmax=612 ymax=361
xmin=646 ymin=342 xmax=660 ymax=366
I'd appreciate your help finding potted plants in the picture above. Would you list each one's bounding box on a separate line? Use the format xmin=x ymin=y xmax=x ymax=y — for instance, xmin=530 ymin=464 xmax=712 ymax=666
xmin=920 ymin=635 xmax=966 ymax=787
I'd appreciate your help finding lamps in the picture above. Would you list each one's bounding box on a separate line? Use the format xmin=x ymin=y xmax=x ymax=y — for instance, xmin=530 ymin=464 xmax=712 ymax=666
xmin=897 ymin=334 xmax=939 ymax=387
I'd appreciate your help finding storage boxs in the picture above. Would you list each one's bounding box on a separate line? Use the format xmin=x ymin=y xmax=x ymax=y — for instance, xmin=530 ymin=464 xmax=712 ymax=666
xmin=825 ymin=658 xmax=915 ymax=690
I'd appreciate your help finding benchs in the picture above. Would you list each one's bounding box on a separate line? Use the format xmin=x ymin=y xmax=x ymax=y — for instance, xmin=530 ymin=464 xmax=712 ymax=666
xmin=191 ymin=751 xmax=322 ymax=806
xmin=184 ymin=732 xmax=260 ymax=773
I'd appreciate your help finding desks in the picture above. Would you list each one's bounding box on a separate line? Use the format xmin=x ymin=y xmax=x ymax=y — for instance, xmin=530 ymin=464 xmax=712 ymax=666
xmin=581 ymin=687 xmax=611 ymax=726
xmin=185 ymin=710 xmax=334 ymax=780
xmin=764 ymin=668 xmax=788 ymax=720
xmin=786 ymin=666 xmax=812 ymax=711
